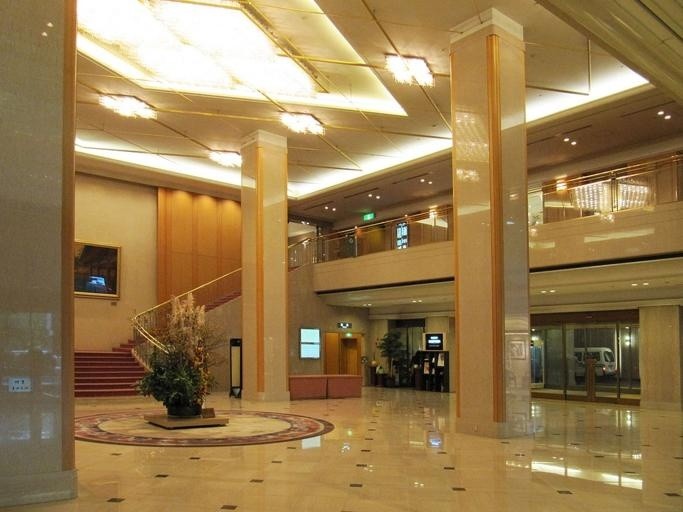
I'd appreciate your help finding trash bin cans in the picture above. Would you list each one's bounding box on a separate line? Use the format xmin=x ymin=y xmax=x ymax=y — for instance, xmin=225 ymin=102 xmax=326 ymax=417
xmin=414 ymin=367 xmax=424 ymax=391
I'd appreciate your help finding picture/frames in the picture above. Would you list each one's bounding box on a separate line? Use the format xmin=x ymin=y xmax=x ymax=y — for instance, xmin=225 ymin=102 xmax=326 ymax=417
xmin=72 ymin=237 xmax=123 ymax=301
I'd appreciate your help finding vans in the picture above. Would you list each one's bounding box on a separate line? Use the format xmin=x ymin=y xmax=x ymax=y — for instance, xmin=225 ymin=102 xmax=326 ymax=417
xmin=574 ymin=343 xmax=617 ymax=379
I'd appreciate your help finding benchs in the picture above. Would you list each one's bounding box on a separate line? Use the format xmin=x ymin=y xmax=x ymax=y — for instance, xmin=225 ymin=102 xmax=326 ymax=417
xmin=288 ymin=372 xmax=366 ymax=401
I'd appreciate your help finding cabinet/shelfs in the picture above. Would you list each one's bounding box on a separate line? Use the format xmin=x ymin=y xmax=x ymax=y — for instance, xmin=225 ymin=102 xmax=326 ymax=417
xmin=414 ymin=349 xmax=449 ymax=391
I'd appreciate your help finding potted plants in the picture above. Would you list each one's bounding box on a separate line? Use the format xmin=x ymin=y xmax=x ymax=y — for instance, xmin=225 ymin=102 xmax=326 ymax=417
xmin=135 ymin=288 xmax=224 ymax=420
xmin=373 ymin=330 xmax=410 ymax=387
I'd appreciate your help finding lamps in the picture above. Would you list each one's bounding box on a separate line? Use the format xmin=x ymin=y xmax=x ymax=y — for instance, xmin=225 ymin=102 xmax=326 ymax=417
xmin=276 ymin=109 xmax=326 ymax=138
xmin=70 ymin=1 xmax=338 ymax=105
xmin=209 ymin=148 xmax=241 ymax=169
xmin=94 ymin=94 xmax=160 ymax=123
xmin=383 ymin=53 xmax=439 ymax=89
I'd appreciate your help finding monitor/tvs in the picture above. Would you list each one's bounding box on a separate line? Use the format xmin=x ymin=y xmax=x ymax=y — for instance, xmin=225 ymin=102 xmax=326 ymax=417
xmin=422 ymin=332 xmax=446 ymax=351
